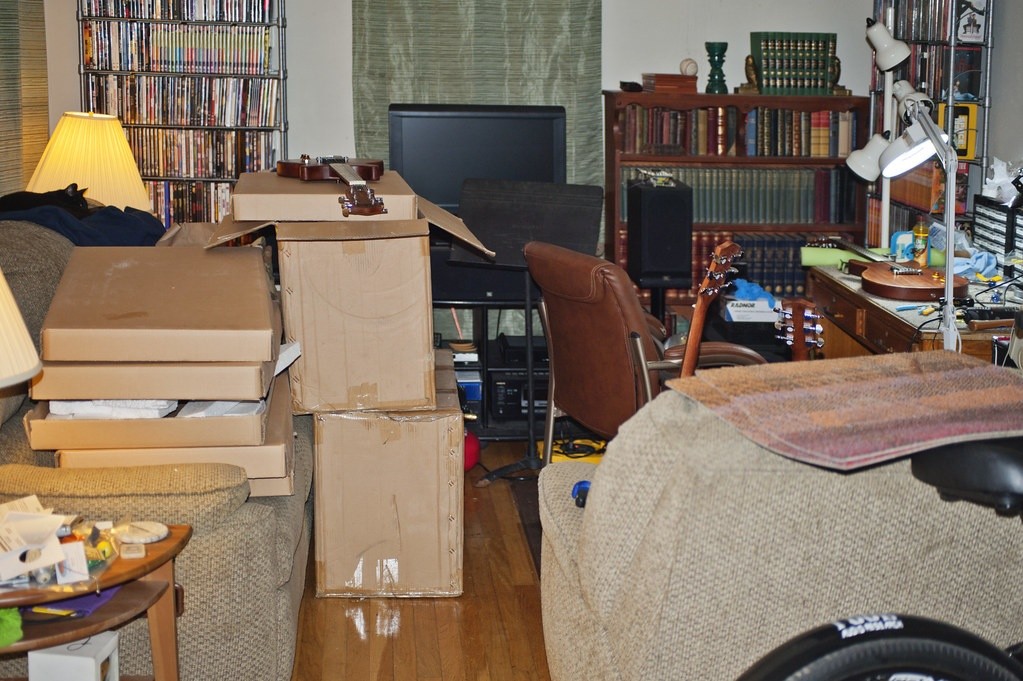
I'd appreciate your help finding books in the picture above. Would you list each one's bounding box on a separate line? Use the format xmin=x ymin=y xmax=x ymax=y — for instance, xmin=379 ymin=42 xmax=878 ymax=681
xmin=750 ymin=32 xmax=836 ymax=95
xmin=80 ymin=0 xmax=278 ymax=248
xmin=618 ymin=104 xmax=856 ymax=158
xmin=864 ymin=0 xmax=987 ymax=247
xmin=618 ymin=230 xmax=856 ymax=337
xmin=620 ymin=165 xmax=855 ymax=224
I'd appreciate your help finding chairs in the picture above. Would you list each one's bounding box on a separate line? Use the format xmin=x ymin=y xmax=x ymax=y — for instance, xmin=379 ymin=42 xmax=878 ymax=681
xmin=526 ymin=241 xmax=767 ymax=470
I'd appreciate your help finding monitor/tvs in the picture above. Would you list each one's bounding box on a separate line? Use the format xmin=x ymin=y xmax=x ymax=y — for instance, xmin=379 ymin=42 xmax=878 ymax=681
xmin=388 ymin=103 xmax=567 ymax=214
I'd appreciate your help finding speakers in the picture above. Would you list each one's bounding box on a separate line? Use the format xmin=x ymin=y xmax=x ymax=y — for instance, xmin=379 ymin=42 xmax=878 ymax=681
xmin=627 ymin=177 xmax=692 ymax=288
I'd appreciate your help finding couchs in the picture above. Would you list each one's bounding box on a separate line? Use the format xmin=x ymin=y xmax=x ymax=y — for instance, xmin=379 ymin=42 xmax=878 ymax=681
xmin=0 ymin=217 xmax=314 ymax=681
xmin=537 ymin=351 xmax=1023 ymax=681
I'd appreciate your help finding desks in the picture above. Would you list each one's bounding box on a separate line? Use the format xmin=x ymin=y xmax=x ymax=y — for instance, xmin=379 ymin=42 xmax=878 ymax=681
xmin=808 ymin=264 xmax=1010 ymax=366
xmin=0 ymin=525 xmax=193 ymax=681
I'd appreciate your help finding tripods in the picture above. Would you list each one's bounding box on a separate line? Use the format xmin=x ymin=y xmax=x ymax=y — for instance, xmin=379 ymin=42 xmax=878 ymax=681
xmin=448 ymin=177 xmax=603 ymax=488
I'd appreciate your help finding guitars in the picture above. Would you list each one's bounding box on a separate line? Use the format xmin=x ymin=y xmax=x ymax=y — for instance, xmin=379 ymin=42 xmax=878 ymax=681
xmin=773 ymin=297 xmax=825 ymax=362
xmin=277 ymin=154 xmax=388 ymax=218
xmin=679 ymin=240 xmax=745 ymax=380
xmin=815 ymin=231 xmax=970 ymax=302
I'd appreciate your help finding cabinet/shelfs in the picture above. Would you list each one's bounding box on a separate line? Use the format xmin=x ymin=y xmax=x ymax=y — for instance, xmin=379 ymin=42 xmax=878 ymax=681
xmin=859 ymin=0 xmax=993 ymax=254
xmin=76 ymin=0 xmax=289 ymax=230
xmin=602 ymin=89 xmax=870 ymax=318
xmin=433 ymin=298 xmax=596 ymax=442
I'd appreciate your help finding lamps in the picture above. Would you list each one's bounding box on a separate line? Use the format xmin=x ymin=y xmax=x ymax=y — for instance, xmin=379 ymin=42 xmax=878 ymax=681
xmin=25 ymin=109 xmax=149 ymax=212
xmin=1 ymin=268 xmax=44 ymax=388
xmin=847 ymin=6 xmax=962 ymax=352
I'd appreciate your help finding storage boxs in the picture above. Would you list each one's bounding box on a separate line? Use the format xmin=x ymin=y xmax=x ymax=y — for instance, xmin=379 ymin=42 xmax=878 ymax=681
xmin=717 ymin=296 xmax=784 ymax=322
xmin=24 ymin=169 xmax=496 ymax=598
xmin=28 ymin=630 xmax=119 ymax=681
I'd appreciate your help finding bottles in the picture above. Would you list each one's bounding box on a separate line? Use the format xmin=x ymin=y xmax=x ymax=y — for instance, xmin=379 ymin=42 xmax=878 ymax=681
xmin=912 ymin=216 xmax=928 ymax=267
